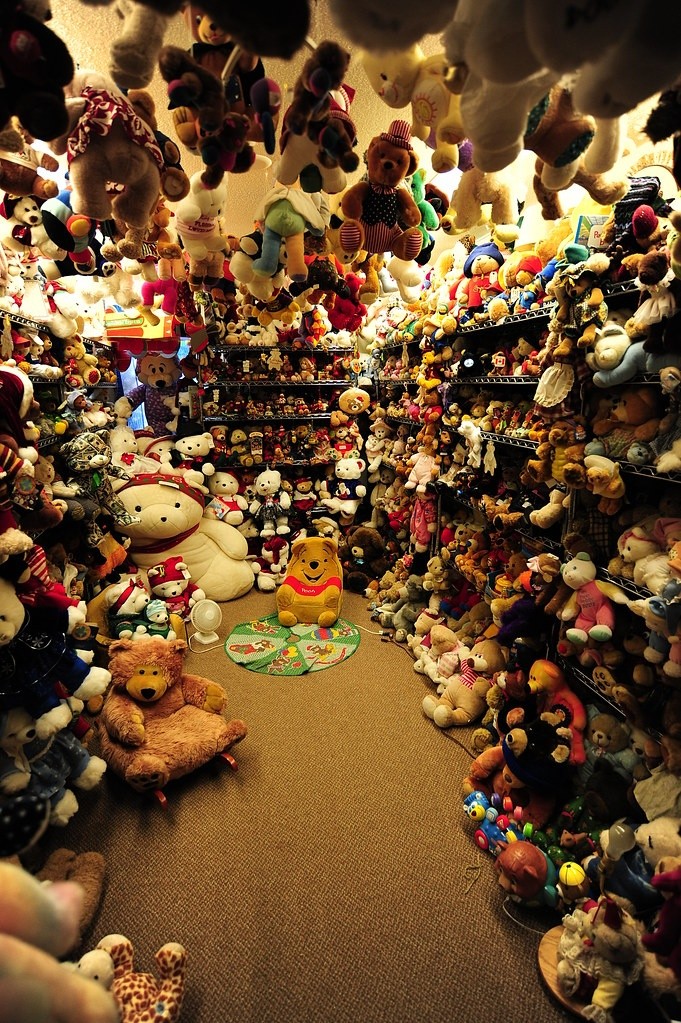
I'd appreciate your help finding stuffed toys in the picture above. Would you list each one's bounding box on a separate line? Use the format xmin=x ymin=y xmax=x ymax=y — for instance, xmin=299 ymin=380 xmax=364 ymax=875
xmin=0 ymin=0 xmax=681 ymax=1023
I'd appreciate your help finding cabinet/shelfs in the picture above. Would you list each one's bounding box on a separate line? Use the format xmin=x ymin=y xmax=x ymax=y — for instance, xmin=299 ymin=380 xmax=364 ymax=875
xmin=0 ymin=309 xmax=120 ymax=538
xmin=207 ymin=270 xmax=681 ymax=721
